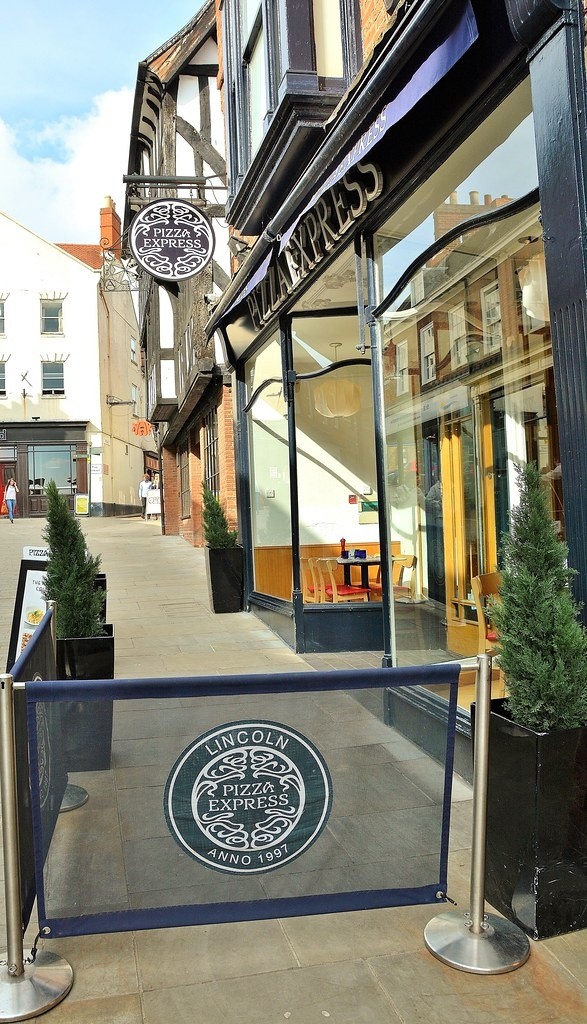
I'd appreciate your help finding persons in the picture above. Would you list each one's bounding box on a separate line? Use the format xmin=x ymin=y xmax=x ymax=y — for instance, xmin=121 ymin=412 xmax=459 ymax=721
xmin=4 ymin=478 xmax=19 ymax=523
xmin=392 ymin=470 xmax=445 ymax=584
xmin=138 ymin=474 xmax=158 ymax=520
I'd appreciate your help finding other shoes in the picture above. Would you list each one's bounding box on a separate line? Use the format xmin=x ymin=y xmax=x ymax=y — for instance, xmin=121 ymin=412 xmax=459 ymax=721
xmin=141 ymin=515 xmax=145 ymax=519
xmin=11 ymin=519 xmax=13 ymax=523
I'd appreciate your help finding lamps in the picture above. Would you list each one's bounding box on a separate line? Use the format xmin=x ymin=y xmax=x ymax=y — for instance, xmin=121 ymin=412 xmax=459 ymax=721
xmin=313 ymin=342 xmax=361 ymax=419
xmin=517 ymin=251 xmax=551 ymax=322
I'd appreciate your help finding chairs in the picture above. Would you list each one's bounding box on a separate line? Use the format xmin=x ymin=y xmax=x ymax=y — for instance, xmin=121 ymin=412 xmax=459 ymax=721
xmin=470 ymin=566 xmax=505 ymax=698
xmin=300 ymin=553 xmax=417 ymax=604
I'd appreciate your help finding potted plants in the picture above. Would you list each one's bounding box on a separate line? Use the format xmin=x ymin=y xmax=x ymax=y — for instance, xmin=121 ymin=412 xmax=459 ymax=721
xmin=38 ymin=477 xmax=115 ymax=771
xmin=470 ymin=460 xmax=587 ymax=941
xmin=199 ymin=479 xmax=244 ymax=613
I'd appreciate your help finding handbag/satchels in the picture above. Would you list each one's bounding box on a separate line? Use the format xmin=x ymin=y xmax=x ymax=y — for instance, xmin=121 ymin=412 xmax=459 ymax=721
xmin=1 ymin=502 xmax=8 ymax=514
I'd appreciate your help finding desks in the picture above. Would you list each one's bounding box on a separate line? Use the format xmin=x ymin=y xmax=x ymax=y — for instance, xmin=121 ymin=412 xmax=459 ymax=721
xmin=315 ymin=557 xmax=407 ymax=602
